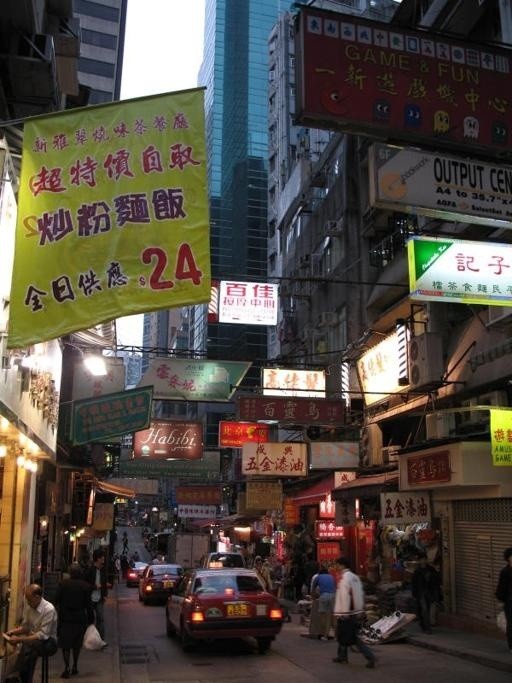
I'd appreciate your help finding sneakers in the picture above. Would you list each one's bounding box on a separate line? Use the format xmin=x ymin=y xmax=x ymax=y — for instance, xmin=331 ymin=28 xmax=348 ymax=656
xmin=61 ymin=667 xmax=70 ymax=677
xmin=71 ymin=666 xmax=79 ymax=675
xmin=366 ymin=656 xmax=376 ymax=668
xmin=5 ymin=671 xmax=23 ymax=683
xmin=332 ymin=656 xmax=349 ymax=664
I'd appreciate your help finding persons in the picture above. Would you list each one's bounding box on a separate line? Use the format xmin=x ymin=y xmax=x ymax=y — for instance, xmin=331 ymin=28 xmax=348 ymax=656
xmin=87 ymin=549 xmax=113 ymax=650
xmin=332 ymin=555 xmax=380 ymax=668
xmin=111 ymin=526 xmax=166 ymax=584
xmin=2 ymin=582 xmax=60 ymax=682
xmin=410 ymin=551 xmax=445 ymax=633
xmin=313 ymin=566 xmax=336 ymax=639
xmin=301 ymin=567 xmax=319 ymax=635
xmin=52 ymin=561 xmax=96 ymax=678
xmin=253 ymin=554 xmax=309 ymax=624
xmin=74 ymin=547 xmax=94 ymax=579
xmin=492 ymin=546 xmax=512 ymax=655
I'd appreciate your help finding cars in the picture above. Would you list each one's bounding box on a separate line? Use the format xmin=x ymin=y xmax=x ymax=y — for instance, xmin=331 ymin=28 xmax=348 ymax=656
xmin=114 ymin=511 xmax=148 ymax=526
xmin=163 ymin=564 xmax=284 ymax=652
xmin=125 ymin=532 xmax=248 ymax=605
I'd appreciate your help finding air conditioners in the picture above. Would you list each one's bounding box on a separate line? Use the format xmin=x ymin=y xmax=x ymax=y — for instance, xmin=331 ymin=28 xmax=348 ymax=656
xmin=488 ymin=305 xmax=512 ymax=322
xmin=478 ymin=389 xmax=509 ymax=423
xmin=407 ymin=332 xmax=443 ymax=390
xmin=323 ymin=221 xmax=341 ymax=233
xmin=380 ymin=445 xmax=401 ymax=465
xmin=318 ymin=312 xmax=338 ymax=327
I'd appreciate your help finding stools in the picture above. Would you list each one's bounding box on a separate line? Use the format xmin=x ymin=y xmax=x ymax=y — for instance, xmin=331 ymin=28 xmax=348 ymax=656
xmin=38 ymin=647 xmax=57 ymax=683
